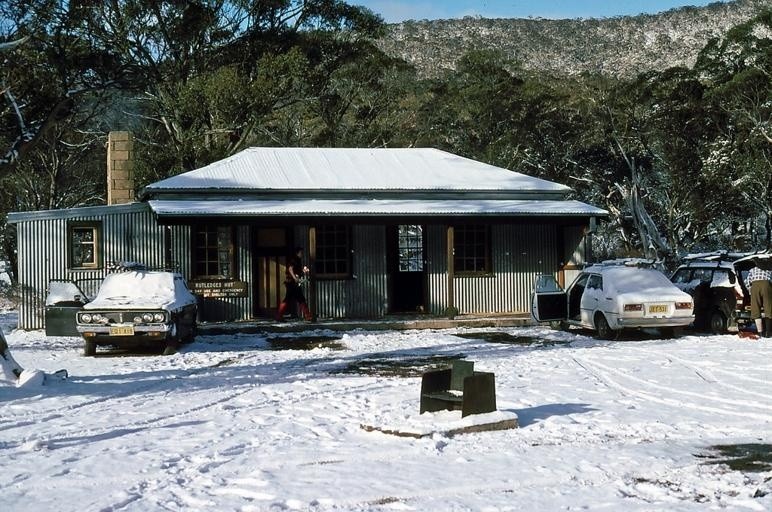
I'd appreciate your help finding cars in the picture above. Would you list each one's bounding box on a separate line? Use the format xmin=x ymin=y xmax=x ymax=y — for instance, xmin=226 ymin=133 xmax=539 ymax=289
xmin=530 ymin=262 xmax=695 ymax=340
xmin=45 ymin=268 xmax=199 ymax=356
xmin=669 ymin=253 xmax=772 ymax=334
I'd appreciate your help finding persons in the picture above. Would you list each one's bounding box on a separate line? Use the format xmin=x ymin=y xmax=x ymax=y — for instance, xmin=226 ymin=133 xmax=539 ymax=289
xmin=741 ymin=259 xmax=771 ymax=339
xmin=274 ymin=247 xmax=318 ymax=323
